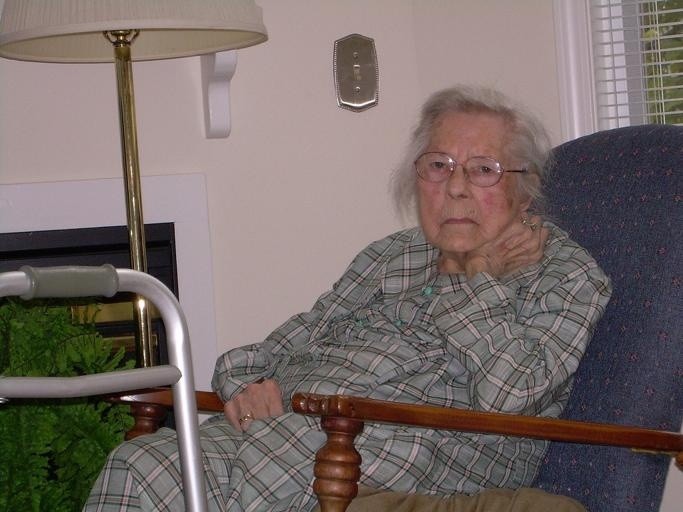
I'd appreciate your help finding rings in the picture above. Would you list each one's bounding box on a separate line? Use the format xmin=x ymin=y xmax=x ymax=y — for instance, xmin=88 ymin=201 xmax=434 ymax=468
xmin=238 ymin=411 xmax=254 ymax=426
xmin=522 ymin=217 xmax=537 ymax=231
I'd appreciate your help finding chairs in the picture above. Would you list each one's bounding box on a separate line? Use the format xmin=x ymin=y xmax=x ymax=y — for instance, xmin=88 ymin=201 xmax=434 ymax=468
xmin=101 ymin=120 xmax=683 ymax=511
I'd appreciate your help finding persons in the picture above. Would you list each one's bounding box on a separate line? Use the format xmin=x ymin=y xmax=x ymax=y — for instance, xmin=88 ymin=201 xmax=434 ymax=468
xmin=81 ymin=82 xmax=614 ymax=512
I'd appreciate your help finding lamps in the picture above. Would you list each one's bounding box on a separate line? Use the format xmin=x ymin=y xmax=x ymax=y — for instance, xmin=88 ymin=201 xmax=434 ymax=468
xmin=0 ymin=0 xmax=268 ymax=367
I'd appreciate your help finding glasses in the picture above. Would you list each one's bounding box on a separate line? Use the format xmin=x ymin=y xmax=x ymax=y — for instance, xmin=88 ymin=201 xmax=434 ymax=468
xmin=412 ymin=149 xmax=524 ymax=192
